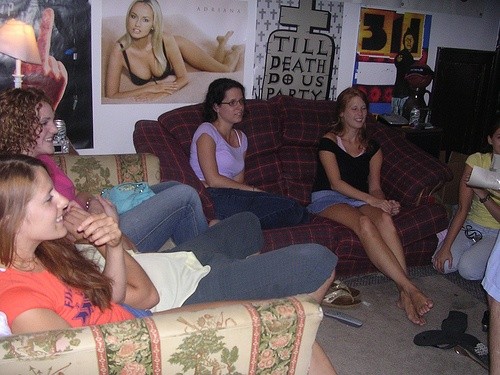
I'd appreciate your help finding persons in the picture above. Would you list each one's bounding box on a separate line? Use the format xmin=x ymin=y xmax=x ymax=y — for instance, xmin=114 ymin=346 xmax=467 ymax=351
xmin=432 ymin=125 xmax=500 ymax=281
xmin=465 ymin=167 xmax=500 ymax=375
xmin=0 ymin=77 xmax=336 ymax=375
xmin=106 ymin=0 xmax=243 ymax=100
xmin=308 ymin=88 xmax=432 ymax=324
xmin=0 ymin=0 xmax=68 ymax=113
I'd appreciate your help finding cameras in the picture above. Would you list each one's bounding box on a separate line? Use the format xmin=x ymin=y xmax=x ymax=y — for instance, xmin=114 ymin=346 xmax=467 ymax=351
xmin=52 ymin=141 xmax=70 ymax=154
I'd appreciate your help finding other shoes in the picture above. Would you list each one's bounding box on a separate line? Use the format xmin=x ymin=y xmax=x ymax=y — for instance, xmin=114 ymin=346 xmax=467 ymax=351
xmin=414 ymin=330 xmax=455 ymax=349
xmin=454 ymin=332 xmax=490 ymax=370
xmin=483 ymin=311 xmax=491 ymax=331
xmin=442 ymin=311 xmax=468 ymax=332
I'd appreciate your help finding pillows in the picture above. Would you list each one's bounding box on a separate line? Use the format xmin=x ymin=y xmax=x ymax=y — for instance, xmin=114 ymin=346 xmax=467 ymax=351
xmin=75 ymin=243 xmax=211 ymax=314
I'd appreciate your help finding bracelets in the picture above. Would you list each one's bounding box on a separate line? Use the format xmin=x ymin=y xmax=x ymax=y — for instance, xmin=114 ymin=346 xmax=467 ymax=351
xmin=480 ymin=190 xmax=490 ymax=203
xmin=85 ymin=196 xmax=93 ymax=211
xmin=252 ymin=184 xmax=254 ymax=191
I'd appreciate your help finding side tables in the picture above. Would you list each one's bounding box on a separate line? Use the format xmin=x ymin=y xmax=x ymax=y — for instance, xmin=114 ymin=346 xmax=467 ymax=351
xmin=390 ymin=127 xmax=454 ymax=163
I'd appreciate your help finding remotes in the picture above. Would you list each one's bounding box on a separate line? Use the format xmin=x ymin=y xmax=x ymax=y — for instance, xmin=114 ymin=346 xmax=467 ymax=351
xmin=324 ymin=309 xmax=363 ymax=328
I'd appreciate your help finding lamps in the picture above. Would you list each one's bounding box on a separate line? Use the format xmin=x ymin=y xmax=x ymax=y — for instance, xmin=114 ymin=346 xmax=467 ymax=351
xmin=0 ymin=18 xmax=42 ymax=88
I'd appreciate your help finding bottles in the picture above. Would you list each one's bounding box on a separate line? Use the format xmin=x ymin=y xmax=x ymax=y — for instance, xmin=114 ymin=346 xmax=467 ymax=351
xmin=402 ymin=86 xmax=431 ymax=129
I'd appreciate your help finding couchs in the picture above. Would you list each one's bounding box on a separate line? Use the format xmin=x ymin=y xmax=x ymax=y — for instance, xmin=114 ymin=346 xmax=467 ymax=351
xmin=132 ymin=95 xmax=455 ymax=276
xmin=0 ymin=151 xmax=323 ymax=375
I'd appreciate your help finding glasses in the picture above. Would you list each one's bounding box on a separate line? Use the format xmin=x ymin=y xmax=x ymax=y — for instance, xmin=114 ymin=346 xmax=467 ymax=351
xmin=220 ymin=99 xmax=244 ymax=105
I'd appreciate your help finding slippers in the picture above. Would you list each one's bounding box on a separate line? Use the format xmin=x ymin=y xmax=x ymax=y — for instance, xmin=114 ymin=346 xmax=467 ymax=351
xmin=322 ymin=289 xmax=362 ymax=308
xmin=330 ymin=280 xmax=361 ymax=298
